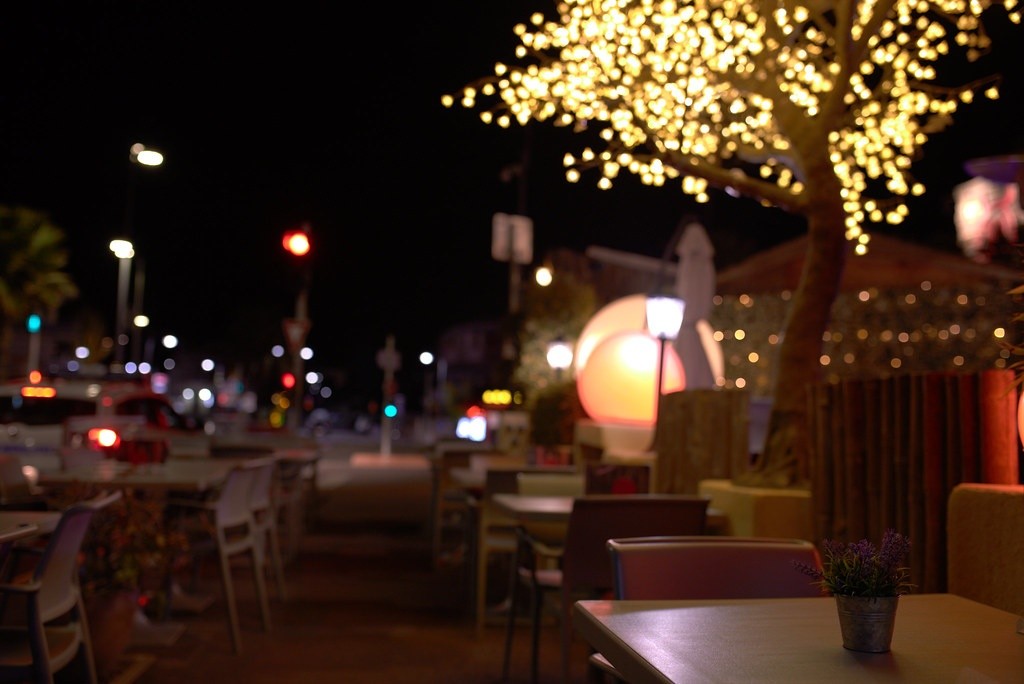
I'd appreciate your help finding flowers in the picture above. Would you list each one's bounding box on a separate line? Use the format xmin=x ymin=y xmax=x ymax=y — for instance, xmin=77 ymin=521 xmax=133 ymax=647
xmin=794 ymin=528 xmax=918 ymax=596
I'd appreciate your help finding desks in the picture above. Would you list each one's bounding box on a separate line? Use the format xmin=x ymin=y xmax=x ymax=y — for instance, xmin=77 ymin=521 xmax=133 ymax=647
xmin=698 ymin=478 xmax=814 ymax=543
xmin=22 ymin=458 xmax=243 ymax=622
xmin=573 ymin=592 xmax=1024 ymax=684
xmin=0 ymin=511 xmax=63 ymax=683
xmin=491 ymin=494 xmax=724 ymax=684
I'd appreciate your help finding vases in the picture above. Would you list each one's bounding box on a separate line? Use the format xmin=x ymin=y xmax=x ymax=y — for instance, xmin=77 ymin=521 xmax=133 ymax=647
xmin=834 ymin=592 xmax=900 ymax=652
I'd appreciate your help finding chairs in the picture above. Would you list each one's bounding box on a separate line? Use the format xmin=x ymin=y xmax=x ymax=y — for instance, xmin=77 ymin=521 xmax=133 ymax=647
xmin=0 ymin=449 xmax=321 ymax=684
xmin=432 ymin=444 xmax=829 ymax=684
